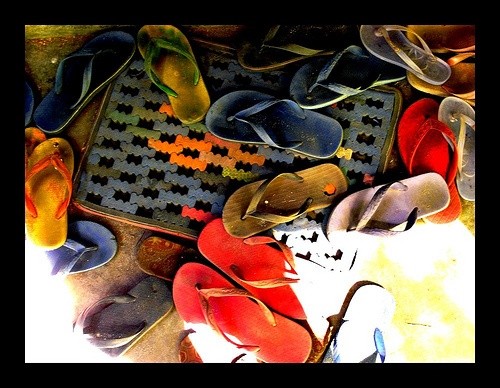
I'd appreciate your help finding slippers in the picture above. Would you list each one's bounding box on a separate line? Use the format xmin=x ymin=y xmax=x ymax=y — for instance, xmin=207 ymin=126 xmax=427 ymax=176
xmin=440 ymin=96 xmax=475 ymax=201
xmin=205 ymin=90 xmax=344 ymax=159
xmin=328 ymin=171 xmax=449 ymax=244
xmin=39 ymin=220 xmax=117 ymax=279
xmin=238 ymin=26 xmax=358 ymax=73
xmin=33 ymin=32 xmax=136 ymax=133
xmin=221 ymin=164 xmax=347 ymax=237
xmin=135 ymin=237 xmax=200 ymax=282
xmin=72 ymin=276 xmax=175 ymax=358
xmin=397 ymin=98 xmax=462 ymax=223
xmin=136 ymin=25 xmax=212 ymax=123
xmin=25 ymin=127 xmax=74 ymax=250
xmin=174 ymin=220 xmax=313 ymax=364
xmin=291 ymin=45 xmax=406 ymax=108
xmin=324 ymin=284 xmax=395 ymax=363
xmin=360 ymin=26 xmax=476 ymax=105
